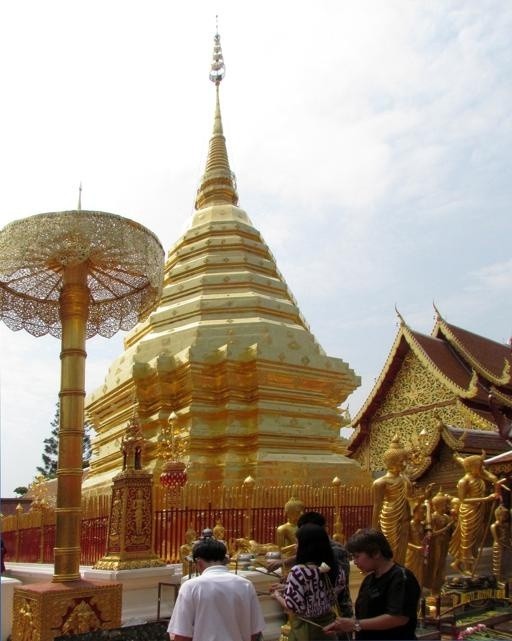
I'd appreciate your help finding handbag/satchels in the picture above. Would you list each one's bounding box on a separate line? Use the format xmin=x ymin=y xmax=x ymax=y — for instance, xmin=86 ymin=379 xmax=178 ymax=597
xmin=280 ymin=609 xmax=310 ymax=640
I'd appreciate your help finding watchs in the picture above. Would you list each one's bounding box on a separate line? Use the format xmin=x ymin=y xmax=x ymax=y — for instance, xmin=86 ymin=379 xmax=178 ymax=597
xmin=353 ymin=618 xmax=362 ymax=632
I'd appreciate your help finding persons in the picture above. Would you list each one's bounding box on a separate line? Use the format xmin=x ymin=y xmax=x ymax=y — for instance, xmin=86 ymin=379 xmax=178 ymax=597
xmin=178 ymin=527 xmax=198 ymax=575
xmin=431 ymin=491 xmax=456 ymax=588
xmin=404 ymin=499 xmax=426 ymax=592
xmin=267 ymin=511 xmax=354 ymax=641
xmin=213 ymin=518 xmax=229 ymax=555
xmin=269 ymin=523 xmax=342 ymax=641
xmin=321 ymin=528 xmax=422 ymax=641
xmin=165 ymin=538 xmax=267 ymax=641
xmin=450 ymin=448 xmax=499 ymax=577
xmin=275 ymin=495 xmax=305 ymax=579
xmin=489 ymin=503 xmax=512 ymax=579
xmin=372 ymin=434 xmax=436 ymax=566
